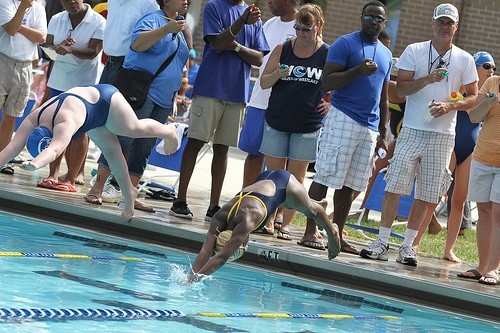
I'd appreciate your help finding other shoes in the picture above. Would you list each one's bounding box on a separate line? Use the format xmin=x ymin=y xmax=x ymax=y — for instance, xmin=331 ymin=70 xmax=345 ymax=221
xmin=0 ymin=164 xmax=15 ymax=175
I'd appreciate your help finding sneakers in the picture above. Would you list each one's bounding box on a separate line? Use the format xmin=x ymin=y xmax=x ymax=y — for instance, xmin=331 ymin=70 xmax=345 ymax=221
xmin=396 ymin=242 xmax=417 ymax=267
xmin=359 ymin=236 xmax=390 ymax=261
xmin=206 ymin=206 xmax=222 ymax=220
xmin=169 ymin=197 xmax=193 ymax=219
xmin=101 ymin=184 xmax=124 ymax=203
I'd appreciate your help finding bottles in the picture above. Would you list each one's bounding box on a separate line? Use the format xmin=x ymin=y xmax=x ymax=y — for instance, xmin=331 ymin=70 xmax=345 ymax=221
xmin=449 ymin=92 xmax=463 ymax=102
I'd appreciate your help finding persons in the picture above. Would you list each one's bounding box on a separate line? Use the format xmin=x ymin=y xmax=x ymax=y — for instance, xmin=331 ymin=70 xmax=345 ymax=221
xmin=298 ymin=0 xmax=392 ymax=255
xmin=314 ymin=31 xmax=497 ymax=264
xmin=359 ymin=3 xmax=479 ymax=267
xmin=169 ymin=0 xmax=334 ymax=242
xmin=185 ymin=169 xmax=342 ymax=286
xmin=469 ymin=75 xmax=500 ymax=285
xmin=0 ymin=0 xmax=201 ymax=213
xmin=0 ymin=84 xmax=180 ymax=222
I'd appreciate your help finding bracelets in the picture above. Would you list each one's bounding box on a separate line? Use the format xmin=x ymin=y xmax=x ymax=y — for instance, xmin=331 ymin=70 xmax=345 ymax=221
xmin=228 ymin=24 xmax=239 ymax=38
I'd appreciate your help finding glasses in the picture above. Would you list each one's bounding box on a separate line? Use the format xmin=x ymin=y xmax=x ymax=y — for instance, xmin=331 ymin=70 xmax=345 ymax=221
xmin=437 ymin=57 xmax=445 ymax=69
xmin=363 ymin=15 xmax=386 ymax=22
xmin=293 ymin=24 xmax=315 ymax=32
xmin=478 ymin=63 xmax=496 ymax=71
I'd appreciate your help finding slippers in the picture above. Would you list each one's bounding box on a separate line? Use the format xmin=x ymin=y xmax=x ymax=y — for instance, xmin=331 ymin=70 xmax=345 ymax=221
xmin=84 ymin=192 xmax=102 ymax=205
xmin=297 ymin=241 xmax=325 ymax=250
xmin=277 ymin=229 xmax=292 ymax=240
xmin=37 ymin=178 xmax=76 ymax=192
xmin=273 ymin=222 xmax=282 ymax=229
xmin=325 ymin=242 xmax=358 ymax=254
xmin=135 ymin=203 xmax=156 ymax=213
xmin=457 ymin=269 xmax=500 ymax=285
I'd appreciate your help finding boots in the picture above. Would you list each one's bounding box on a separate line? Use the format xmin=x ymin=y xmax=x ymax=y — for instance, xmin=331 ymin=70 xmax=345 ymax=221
xmin=252 ymin=227 xmax=274 ymax=235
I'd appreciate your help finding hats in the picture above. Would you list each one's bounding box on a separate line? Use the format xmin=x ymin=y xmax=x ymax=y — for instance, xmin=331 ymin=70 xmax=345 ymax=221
xmin=215 ymin=230 xmax=243 ymax=262
xmin=26 ymin=126 xmax=52 ymax=158
xmin=472 ymin=51 xmax=494 ymax=69
xmin=190 ymin=49 xmax=197 ymax=58
xmin=433 ymin=3 xmax=459 ymax=22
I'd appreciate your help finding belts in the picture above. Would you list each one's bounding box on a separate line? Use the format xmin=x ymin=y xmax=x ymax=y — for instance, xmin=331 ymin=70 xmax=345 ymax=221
xmin=110 ymin=56 xmax=125 ymax=63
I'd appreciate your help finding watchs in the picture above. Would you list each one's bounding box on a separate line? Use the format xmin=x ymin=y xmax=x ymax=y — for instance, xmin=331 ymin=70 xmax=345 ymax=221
xmin=234 ymin=43 xmax=243 ymax=55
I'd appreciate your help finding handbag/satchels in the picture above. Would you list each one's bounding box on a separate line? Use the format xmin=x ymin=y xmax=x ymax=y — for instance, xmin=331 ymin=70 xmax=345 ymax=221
xmin=109 ymin=66 xmax=153 ymax=109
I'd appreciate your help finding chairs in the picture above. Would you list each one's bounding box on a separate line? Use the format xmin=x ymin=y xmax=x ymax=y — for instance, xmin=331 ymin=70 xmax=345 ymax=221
xmin=133 ymin=120 xmax=196 ymax=199
xmin=345 ymin=165 xmax=433 ymax=247
